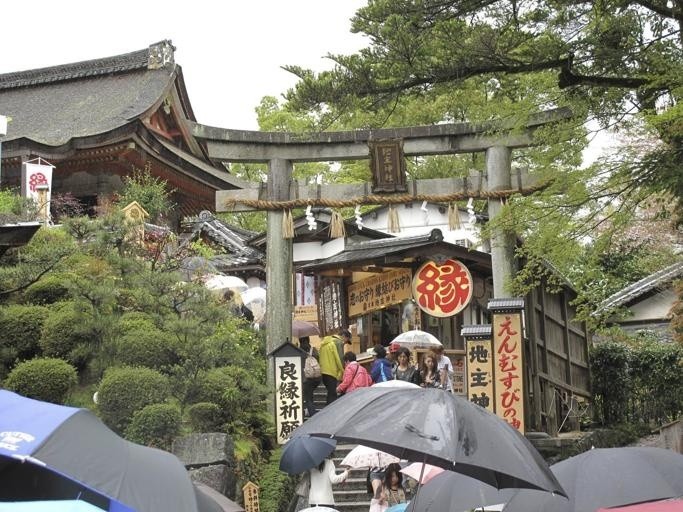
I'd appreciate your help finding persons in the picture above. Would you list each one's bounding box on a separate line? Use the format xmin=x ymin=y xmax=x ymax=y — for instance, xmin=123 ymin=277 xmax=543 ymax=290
xmin=299 ymin=329 xmax=454 ymax=418
xmin=369 ymin=466 xmax=387 ymax=497
xmin=221 ymin=291 xmax=242 ymax=318
xmin=374 ymin=463 xmax=407 ymax=506
xmin=308 ymin=457 xmax=351 ymax=508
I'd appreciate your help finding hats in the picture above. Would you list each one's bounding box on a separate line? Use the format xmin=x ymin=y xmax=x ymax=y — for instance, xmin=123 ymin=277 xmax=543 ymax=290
xmin=389 ymin=343 xmax=400 ymax=353
xmin=366 ymin=347 xmax=378 ymax=356
xmin=338 ymin=329 xmax=353 ymax=345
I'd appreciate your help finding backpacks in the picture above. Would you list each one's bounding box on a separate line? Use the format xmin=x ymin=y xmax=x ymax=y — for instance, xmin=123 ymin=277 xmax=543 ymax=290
xmin=298 ymin=346 xmax=321 ymax=378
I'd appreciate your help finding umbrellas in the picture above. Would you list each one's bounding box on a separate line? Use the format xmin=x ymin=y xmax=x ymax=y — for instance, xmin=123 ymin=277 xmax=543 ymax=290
xmin=207 ymin=275 xmax=249 ymax=289
xmin=291 ymin=320 xmax=320 ymax=337
xmin=240 ymin=286 xmax=266 ymax=305
xmin=192 ymin=480 xmax=246 ymax=512
xmin=0 ymin=390 xmax=197 ymax=512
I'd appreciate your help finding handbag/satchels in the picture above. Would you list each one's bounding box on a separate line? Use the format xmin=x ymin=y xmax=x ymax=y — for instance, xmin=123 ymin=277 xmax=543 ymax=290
xmin=367 ymin=472 xmax=374 ymax=493
xmin=369 ymin=498 xmax=389 ymax=512
xmin=296 ymin=473 xmax=310 ymax=496
